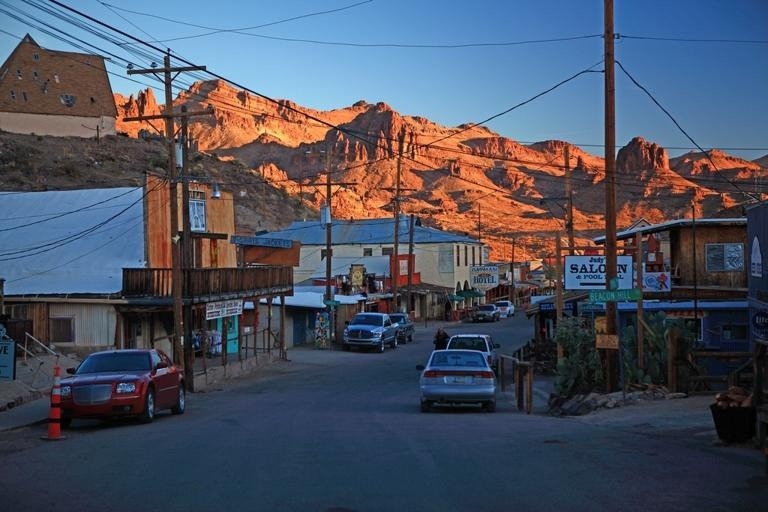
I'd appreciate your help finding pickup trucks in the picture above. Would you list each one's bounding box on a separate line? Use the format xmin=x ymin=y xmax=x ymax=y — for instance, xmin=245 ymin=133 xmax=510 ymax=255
xmin=342 ymin=312 xmax=414 ymax=352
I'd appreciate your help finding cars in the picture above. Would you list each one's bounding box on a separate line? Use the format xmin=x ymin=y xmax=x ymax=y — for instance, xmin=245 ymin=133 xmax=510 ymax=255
xmin=51 ymin=348 xmax=185 ymax=426
xmin=416 ymin=349 xmax=497 ymax=410
xmin=471 ymin=300 xmax=515 ymax=323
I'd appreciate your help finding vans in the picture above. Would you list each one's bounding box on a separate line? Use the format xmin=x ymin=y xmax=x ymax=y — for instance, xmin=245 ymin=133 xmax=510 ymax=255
xmin=445 ymin=334 xmax=500 ymax=366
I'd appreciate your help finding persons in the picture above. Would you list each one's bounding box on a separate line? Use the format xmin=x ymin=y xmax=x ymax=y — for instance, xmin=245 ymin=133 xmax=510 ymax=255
xmin=435 ymin=327 xmax=450 ymax=341
